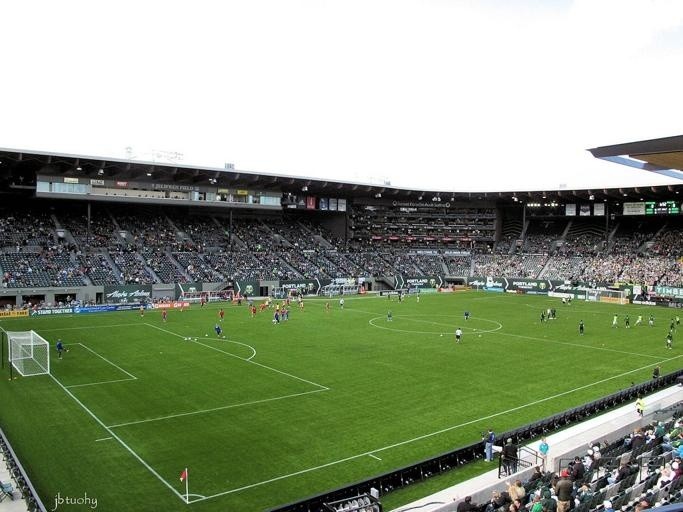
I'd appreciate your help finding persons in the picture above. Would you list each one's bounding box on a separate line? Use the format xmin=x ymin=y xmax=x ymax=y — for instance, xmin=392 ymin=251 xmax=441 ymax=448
xmin=653 ymin=366 xmax=660 ymax=379
xmin=457 ymin=393 xmax=683 ymax=512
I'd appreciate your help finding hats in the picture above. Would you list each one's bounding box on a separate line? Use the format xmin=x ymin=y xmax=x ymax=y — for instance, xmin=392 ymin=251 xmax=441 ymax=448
xmin=506 ymin=438 xmax=512 ymax=442
xmin=532 ymin=444 xmax=680 ymax=512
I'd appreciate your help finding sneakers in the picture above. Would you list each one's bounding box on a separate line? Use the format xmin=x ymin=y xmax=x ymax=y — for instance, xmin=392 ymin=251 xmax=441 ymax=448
xmin=483 ymin=457 xmax=493 ymax=462
